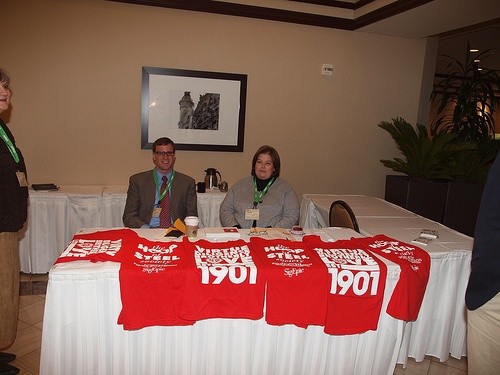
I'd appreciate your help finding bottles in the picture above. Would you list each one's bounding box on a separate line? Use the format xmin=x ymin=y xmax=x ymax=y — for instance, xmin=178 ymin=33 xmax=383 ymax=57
xmin=220 ymin=181 xmax=228 ymax=192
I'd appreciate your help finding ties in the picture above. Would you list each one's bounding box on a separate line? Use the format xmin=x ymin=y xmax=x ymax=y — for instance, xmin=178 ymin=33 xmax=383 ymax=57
xmin=160 ymin=176 xmax=171 ymax=229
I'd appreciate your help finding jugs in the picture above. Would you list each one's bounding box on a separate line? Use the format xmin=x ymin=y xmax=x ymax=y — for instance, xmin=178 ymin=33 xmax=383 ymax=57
xmin=204 ymin=168 xmax=222 ymax=189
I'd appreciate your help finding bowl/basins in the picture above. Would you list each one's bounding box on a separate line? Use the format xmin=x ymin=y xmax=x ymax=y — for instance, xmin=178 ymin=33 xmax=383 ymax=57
xmin=291 ymin=226 xmax=304 ymax=234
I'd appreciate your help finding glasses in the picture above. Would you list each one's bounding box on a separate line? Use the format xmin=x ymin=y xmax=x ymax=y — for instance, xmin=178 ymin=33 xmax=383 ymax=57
xmin=156 ymin=151 xmax=175 ymax=156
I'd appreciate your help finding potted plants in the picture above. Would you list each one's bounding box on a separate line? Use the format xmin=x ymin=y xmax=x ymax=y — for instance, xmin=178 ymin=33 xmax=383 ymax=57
xmin=378 ymin=115 xmax=500 ymax=236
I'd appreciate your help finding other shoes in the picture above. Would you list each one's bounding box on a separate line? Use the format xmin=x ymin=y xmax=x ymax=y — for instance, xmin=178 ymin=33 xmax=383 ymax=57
xmin=0 ymin=353 xmax=20 ymax=364
xmin=0 ymin=364 xmax=20 ymax=375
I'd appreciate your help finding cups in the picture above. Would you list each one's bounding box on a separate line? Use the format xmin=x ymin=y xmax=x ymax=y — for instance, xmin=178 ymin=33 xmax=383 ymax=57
xmin=184 ymin=216 xmax=200 ymax=238
xmin=196 ymin=182 xmax=205 ymax=193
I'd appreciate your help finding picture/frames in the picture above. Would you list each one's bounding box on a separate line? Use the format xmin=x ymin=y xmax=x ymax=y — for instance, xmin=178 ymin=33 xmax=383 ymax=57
xmin=140 ymin=65 xmax=248 ymax=153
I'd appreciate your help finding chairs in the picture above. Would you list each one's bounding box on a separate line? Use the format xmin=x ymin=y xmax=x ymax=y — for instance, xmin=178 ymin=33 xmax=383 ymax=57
xmin=327 ymin=200 xmax=359 ymax=230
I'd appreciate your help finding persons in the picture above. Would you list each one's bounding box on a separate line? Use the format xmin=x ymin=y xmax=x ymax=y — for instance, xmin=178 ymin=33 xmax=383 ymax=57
xmin=219 ymin=145 xmax=299 ymax=229
xmin=0 ymin=68 xmax=29 ymax=375
xmin=465 ymin=153 xmax=500 ymax=375
xmin=122 ymin=137 xmax=198 ymax=229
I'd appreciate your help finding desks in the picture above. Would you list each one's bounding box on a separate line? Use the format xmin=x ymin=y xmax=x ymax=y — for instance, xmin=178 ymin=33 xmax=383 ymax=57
xmin=19 ymin=180 xmax=229 ymax=276
xmin=38 ymin=222 xmax=413 ymax=375
xmin=296 ymin=194 xmax=477 ymax=361
